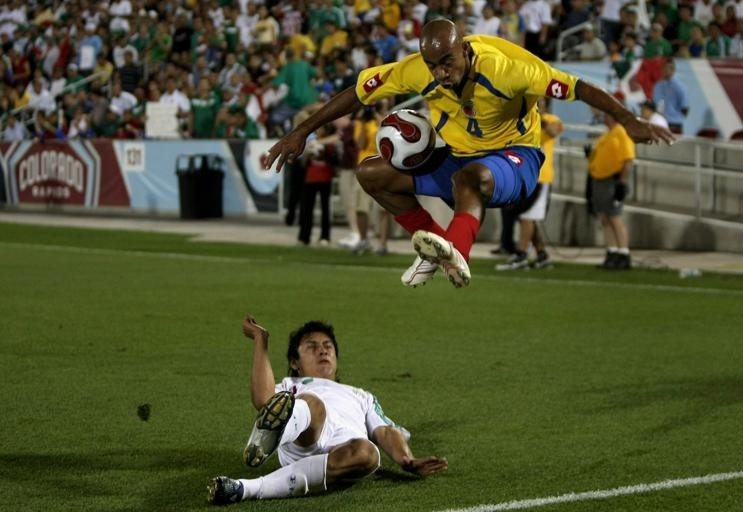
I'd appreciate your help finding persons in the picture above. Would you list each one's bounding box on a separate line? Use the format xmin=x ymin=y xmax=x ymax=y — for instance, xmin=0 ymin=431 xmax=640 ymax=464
xmin=491 ymin=98 xmax=564 ymax=271
xmin=282 ymin=94 xmax=392 ymax=256
xmin=584 ymin=110 xmax=636 ymax=271
xmin=204 ymin=314 xmax=450 ymax=506
xmin=1 ymin=1 xmax=474 ymax=141
xmin=261 ymin=19 xmax=680 ymax=287
xmin=473 ymin=1 xmax=743 ymax=131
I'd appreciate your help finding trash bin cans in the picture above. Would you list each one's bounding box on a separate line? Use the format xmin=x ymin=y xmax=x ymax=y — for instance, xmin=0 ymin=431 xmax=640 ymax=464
xmin=175 ymin=154 xmax=226 ymax=220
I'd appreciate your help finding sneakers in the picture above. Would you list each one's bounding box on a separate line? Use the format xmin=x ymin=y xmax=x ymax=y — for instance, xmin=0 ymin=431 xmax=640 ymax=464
xmin=603 ymin=251 xmax=629 ymax=270
xmin=207 ymin=476 xmax=243 ymax=504
xmin=401 ymin=230 xmax=471 ymax=288
xmin=495 ymin=250 xmax=550 ymax=271
xmin=243 ymin=391 xmax=294 ymax=467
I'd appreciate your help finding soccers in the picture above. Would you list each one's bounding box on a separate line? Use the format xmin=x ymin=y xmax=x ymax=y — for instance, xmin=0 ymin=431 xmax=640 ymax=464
xmin=374 ymin=109 xmax=438 ymax=171
